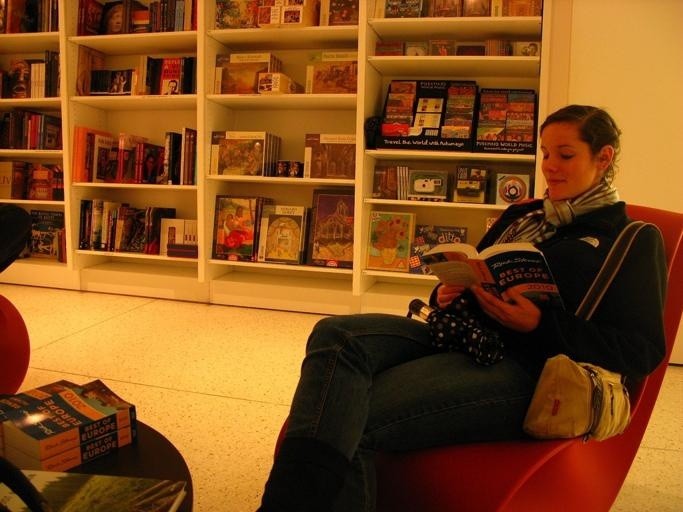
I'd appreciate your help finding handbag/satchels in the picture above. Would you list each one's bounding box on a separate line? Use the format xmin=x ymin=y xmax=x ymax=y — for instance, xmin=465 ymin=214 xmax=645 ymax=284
xmin=527 ymin=357 xmax=633 ymax=442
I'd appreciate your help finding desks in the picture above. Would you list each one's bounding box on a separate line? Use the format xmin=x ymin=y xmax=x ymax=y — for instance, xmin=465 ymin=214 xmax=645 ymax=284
xmin=0 ymin=393 xmax=192 ymax=511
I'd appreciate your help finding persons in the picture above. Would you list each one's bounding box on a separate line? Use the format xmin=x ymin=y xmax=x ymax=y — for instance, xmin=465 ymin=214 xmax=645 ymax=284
xmin=260 ymin=104 xmax=669 ymax=511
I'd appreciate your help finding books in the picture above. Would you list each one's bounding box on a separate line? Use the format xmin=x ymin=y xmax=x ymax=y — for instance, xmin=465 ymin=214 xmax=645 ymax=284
xmin=0 ymin=464 xmax=189 ymax=512
xmin=0 ymin=0 xmax=67 ymax=264
xmin=71 ymin=0 xmax=198 ymax=259
xmin=209 ymin=0 xmax=359 ymax=270
xmin=0 ymin=377 xmax=138 ymax=473
xmin=365 ymin=0 xmax=542 ymax=275
xmin=418 ymin=244 xmax=558 ymax=307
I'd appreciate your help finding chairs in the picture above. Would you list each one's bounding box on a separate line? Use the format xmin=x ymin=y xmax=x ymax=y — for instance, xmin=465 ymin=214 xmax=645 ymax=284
xmin=0 ymin=294 xmax=31 ymax=399
xmin=272 ymin=198 xmax=683 ymax=512
